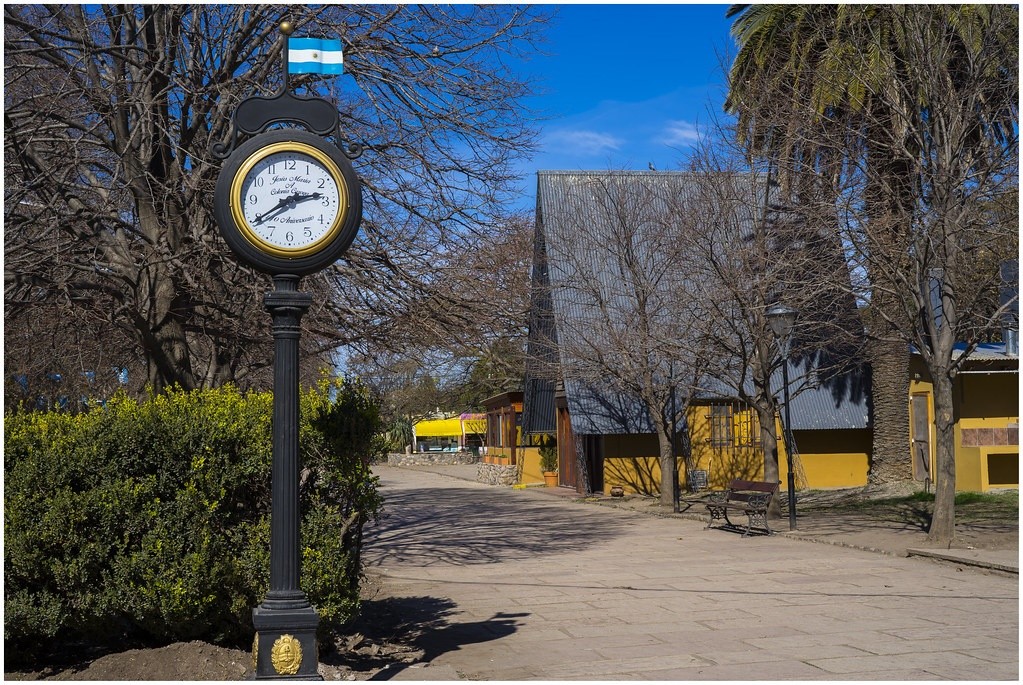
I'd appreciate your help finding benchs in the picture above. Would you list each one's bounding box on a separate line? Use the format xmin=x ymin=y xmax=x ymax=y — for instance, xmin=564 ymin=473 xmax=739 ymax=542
xmin=705 ymin=476 xmax=784 ymax=536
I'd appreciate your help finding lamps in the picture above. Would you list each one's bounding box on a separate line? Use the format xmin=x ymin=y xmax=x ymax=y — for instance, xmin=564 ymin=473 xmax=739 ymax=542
xmin=914 ymin=372 xmax=921 ymax=381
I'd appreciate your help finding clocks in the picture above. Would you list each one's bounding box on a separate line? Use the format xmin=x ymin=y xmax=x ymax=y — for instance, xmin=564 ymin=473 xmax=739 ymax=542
xmin=216 ymin=129 xmax=364 ymax=270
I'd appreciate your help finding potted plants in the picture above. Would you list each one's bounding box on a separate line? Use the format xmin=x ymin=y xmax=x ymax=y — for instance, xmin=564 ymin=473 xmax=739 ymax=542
xmin=537 ymin=439 xmax=559 ymax=488
xmin=485 ymin=453 xmax=493 ymax=464
xmin=498 ymin=455 xmax=508 ymax=466
xmin=490 ymin=454 xmax=499 ymax=464
